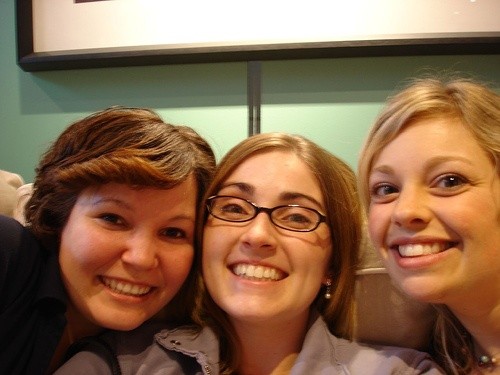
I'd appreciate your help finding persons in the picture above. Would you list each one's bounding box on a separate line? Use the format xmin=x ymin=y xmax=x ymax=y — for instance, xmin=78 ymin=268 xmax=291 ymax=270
xmin=52 ymin=132 xmax=442 ymax=375
xmin=0 ymin=104 xmax=217 ymax=375
xmin=358 ymin=74 xmax=500 ymax=375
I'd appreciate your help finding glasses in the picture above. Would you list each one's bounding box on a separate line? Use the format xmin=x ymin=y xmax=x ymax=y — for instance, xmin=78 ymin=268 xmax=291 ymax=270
xmin=205 ymin=195 xmax=327 ymax=232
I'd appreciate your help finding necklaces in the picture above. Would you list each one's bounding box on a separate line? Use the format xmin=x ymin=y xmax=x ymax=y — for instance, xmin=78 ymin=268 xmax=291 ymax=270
xmin=477 ymin=354 xmax=500 ymax=366
